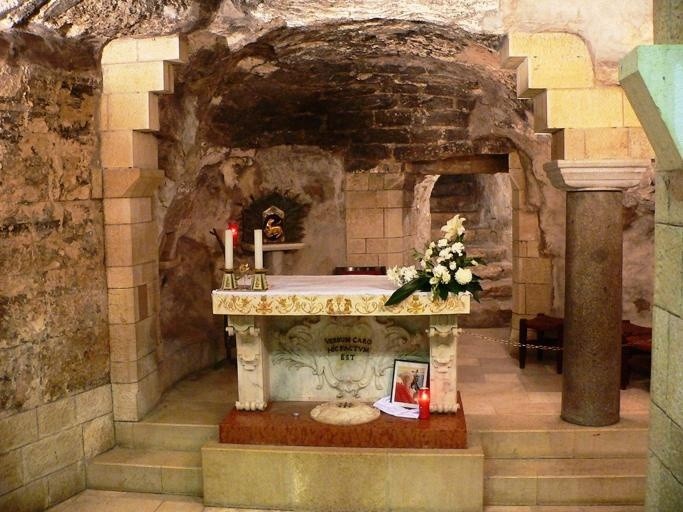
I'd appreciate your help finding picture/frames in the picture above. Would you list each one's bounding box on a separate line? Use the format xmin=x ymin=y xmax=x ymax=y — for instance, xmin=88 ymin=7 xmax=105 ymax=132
xmin=389 ymin=358 xmax=430 ymax=409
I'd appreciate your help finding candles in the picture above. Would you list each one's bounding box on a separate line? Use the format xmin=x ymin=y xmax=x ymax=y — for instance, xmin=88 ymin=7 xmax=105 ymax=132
xmin=253 ymin=228 xmax=263 ymax=269
xmin=223 ymin=228 xmax=234 ymax=271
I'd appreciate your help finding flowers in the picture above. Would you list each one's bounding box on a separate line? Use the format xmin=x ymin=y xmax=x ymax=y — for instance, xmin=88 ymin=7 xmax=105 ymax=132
xmin=381 ymin=208 xmax=489 ymax=313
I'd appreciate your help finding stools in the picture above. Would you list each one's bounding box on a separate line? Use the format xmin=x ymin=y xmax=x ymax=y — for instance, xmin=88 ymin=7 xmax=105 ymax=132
xmin=514 ymin=311 xmax=654 ymax=394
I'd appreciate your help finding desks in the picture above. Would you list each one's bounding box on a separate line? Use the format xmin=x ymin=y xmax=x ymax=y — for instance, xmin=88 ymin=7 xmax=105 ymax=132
xmin=209 ymin=269 xmax=474 ymax=420
